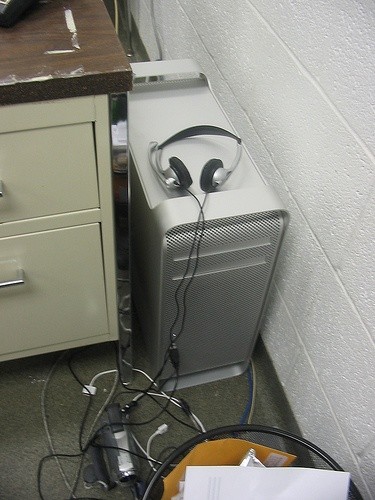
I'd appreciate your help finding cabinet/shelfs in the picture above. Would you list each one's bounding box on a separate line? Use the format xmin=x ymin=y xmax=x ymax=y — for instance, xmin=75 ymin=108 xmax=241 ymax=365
xmin=0 ymin=1 xmax=138 ymax=364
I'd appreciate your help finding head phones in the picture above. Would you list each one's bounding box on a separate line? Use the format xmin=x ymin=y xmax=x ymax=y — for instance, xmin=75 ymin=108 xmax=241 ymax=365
xmin=148 ymin=122 xmax=242 ymax=193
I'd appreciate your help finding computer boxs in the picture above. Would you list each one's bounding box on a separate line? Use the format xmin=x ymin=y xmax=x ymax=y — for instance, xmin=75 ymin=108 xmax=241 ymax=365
xmin=125 ymin=78 xmax=291 ymax=394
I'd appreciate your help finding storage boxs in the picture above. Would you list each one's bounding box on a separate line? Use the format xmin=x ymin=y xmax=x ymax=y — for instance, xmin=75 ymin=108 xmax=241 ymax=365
xmin=141 ymin=423 xmax=364 ymax=500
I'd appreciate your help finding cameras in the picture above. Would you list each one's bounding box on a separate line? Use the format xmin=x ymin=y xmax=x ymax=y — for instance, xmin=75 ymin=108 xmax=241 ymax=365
xmin=90 ymin=404 xmax=141 ymax=491
